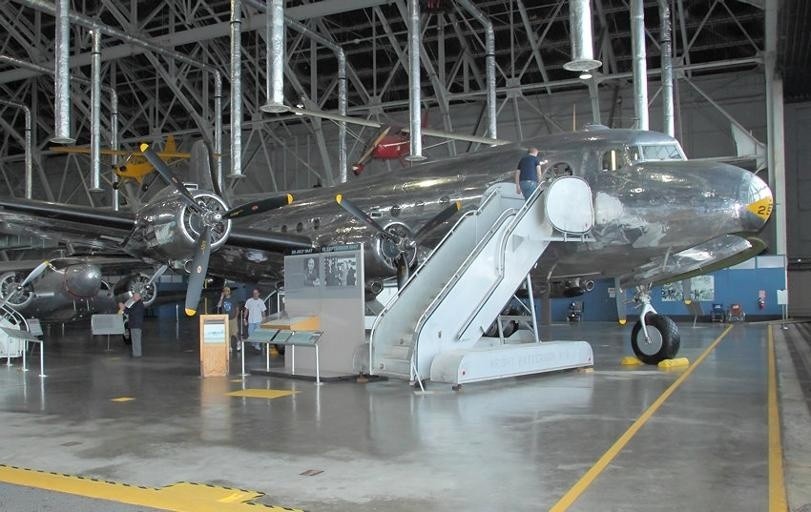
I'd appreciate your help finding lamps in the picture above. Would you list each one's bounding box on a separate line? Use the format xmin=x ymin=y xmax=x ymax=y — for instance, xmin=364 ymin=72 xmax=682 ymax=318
xmin=579 ymin=70 xmax=592 ymax=80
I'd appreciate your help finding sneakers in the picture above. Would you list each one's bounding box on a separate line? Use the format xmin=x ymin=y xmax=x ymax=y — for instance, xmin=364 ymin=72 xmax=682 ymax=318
xmin=229 ymin=339 xmax=263 ymax=354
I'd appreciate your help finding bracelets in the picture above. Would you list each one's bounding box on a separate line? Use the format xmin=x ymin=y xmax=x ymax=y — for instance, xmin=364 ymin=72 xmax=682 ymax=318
xmin=244 ymin=318 xmax=247 ymax=321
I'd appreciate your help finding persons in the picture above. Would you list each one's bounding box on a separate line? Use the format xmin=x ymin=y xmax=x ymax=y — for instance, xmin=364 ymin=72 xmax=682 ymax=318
xmin=217 ymin=287 xmax=243 ymax=353
xmin=118 ymin=292 xmax=146 ymax=358
xmin=243 ymin=289 xmax=268 ymax=352
xmin=306 ymin=257 xmax=320 ymax=288
xmin=515 ymin=145 xmax=544 ymax=207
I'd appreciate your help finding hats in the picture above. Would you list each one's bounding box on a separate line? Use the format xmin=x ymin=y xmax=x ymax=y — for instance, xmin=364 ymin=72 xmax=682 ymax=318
xmin=222 ymin=286 xmax=231 ymax=291
